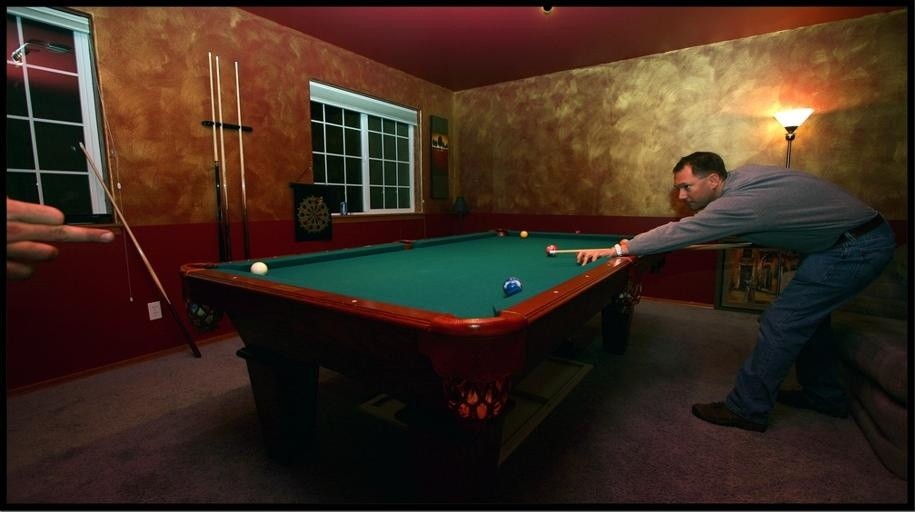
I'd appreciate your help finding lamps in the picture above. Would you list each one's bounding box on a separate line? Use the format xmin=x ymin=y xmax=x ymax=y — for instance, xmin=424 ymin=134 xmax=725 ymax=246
xmin=771 ymin=107 xmax=814 ymax=169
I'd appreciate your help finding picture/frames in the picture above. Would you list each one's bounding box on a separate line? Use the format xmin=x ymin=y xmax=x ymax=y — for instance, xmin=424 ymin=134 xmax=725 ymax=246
xmin=712 ymin=236 xmax=804 ymax=315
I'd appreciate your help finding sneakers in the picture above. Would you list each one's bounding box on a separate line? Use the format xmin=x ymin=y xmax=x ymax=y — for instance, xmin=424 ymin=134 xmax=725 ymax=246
xmin=692 ymin=402 xmax=767 ymax=432
xmin=777 ymin=389 xmax=848 ymax=419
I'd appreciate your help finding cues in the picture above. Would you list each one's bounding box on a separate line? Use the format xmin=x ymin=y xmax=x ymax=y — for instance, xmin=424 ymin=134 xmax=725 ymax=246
xmin=235 ymin=61 xmax=251 ymax=260
xmin=78 ymin=142 xmax=202 ymax=357
xmin=208 ymin=51 xmax=232 ymax=262
xmin=550 ymin=242 xmax=754 ymax=255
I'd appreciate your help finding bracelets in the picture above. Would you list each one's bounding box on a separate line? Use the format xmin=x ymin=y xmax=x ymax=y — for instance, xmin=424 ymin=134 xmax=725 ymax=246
xmin=614 ymin=243 xmax=624 ymax=257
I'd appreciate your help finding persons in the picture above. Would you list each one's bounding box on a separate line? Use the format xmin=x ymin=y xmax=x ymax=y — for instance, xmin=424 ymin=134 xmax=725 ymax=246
xmin=6 ymin=194 xmax=115 ymax=283
xmin=573 ymin=149 xmax=901 ymax=433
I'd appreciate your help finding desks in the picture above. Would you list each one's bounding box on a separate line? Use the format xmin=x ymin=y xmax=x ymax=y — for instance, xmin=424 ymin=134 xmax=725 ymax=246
xmin=178 ymin=227 xmax=667 ymax=506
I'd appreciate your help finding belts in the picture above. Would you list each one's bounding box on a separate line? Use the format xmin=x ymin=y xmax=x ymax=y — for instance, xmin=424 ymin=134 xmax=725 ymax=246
xmin=838 ymin=214 xmax=884 ymax=243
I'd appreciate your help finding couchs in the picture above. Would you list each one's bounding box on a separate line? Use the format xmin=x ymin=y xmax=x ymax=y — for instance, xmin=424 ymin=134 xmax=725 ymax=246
xmin=823 ymin=244 xmax=906 ymax=480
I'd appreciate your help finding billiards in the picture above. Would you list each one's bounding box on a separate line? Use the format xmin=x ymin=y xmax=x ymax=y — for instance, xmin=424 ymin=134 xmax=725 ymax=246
xmin=450 ymin=383 xmax=507 ymax=418
xmin=618 ymin=293 xmax=634 ymax=308
xmin=620 ymin=239 xmax=629 ymax=246
xmin=546 ymin=245 xmax=558 ymax=256
xmin=520 ymin=230 xmax=529 ymax=238
xmin=503 ymin=277 xmax=523 ymax=296
xmin=188 ymin=302 xmax=217 ymax=332
xmin=250 ymin=262 xmax=268 ymax=276
